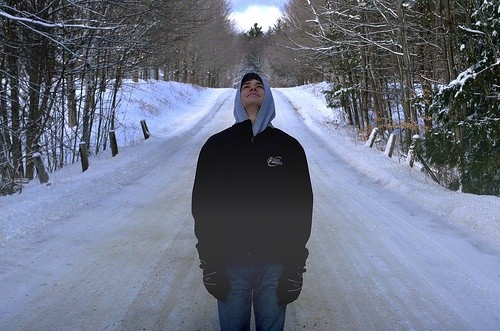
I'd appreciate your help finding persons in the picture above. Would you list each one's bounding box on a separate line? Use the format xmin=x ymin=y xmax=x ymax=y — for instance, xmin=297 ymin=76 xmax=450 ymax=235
xmin=191 ymin=72 xmax=314 ymax=331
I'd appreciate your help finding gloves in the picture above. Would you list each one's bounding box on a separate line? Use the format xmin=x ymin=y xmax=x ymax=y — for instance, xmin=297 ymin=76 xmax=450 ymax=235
xmin=277 ymin=263 xmax=306 ymax=304
xmin=201 ymin=268 xmax=231 ymax=301
